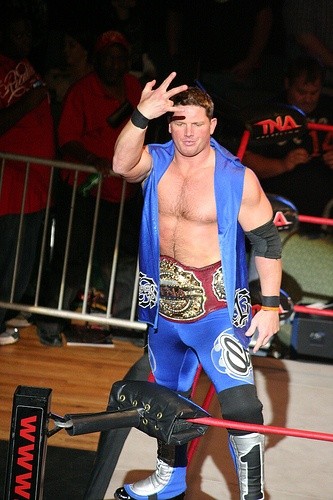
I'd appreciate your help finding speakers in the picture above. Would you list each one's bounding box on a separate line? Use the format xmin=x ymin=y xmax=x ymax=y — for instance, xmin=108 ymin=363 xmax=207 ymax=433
xmin=287 ymin=318 xmax=333 ymax=366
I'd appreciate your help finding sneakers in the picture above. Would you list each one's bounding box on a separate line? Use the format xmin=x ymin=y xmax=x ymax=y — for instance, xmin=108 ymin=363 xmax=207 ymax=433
xmin=0 ymin=328 xmax=20 ymax=345
xmin=4 ymin=311 xmax=34 ymax=326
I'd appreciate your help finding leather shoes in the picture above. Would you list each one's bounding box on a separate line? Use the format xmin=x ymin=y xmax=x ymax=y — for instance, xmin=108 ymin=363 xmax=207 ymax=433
xmin=37 ymin=323 xmax=64 ymax=345
xmin=113 ymin=485 xmax=185 ymax=500
xmin=109 ymin=329 xmax=147 ymax=347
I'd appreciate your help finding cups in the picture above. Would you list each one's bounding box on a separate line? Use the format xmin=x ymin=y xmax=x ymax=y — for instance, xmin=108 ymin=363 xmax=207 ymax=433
xmin=107 ymin=101 xmax=137 ymax=128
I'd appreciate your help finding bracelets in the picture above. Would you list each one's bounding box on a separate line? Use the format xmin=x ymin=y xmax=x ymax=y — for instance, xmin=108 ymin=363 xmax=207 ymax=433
xmin=131 ymin=108 xmax=149 ymax=129
xmin=261 ymin=306 xmax=279 ymax=311
xmin=261 ymin=296 xmax=280 ymax=307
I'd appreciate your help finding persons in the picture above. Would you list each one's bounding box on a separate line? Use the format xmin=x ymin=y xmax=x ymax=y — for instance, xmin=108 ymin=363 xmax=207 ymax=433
xmin=0 ymin=0 xmax=333 ymax=347
xmin=113 ymin=72 xmax=282 ymax=500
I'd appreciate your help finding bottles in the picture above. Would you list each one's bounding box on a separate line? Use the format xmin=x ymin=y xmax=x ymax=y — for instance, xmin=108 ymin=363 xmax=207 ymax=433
xmin=76 ymin=166 xmax=113 ymax=196
